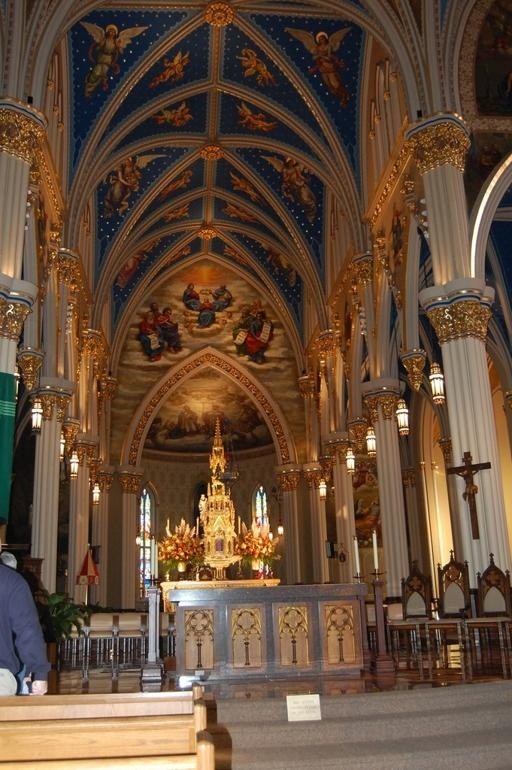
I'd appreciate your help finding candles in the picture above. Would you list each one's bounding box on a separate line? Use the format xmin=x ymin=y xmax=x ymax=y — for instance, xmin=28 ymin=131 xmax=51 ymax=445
xmin=353 ymin=529 xmax=379 ymax=573
xmin=150 ymin=536 xmax=155 ymax=575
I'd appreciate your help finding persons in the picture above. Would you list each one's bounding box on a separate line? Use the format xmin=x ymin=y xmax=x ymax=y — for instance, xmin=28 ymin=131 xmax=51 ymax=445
xmin=230 ymin=307 xmax=254 ymax=355
xmin=177 ymin=404 xmax=199 ymax=432
xmin=249 ymin=310 xmax=273 ymax=364
xmin=239 ymin=44 xmax=281 ymax=93
xmin=0 ymin=561 xmax=52 ymax=698
xmin=355 ymin=492 xmax=382 ymax=547
xmin=146 ymin=101 xmax=195 ymax=130
xmin=201 ymin=404 xmax=224 ymax=433
xmin=234 ymin=104 xmax=281 ymax=134
xmin=198 ymin=297 xmax=215 ymax=328
xmin=149 ymin=302 xmax=162 ymax=317
xmin=283 ymin=156 xmax=320 ymax=221
xmin=213 ymin=284 xmax=232 ymax=312
xmin=250 ymin=298 xmax=264 ymax=316
xmin=76 ymin=17 xmax=126 ymax=103
xmin=183 ymin=281 xmax=200 ymax=310
xmin=145 ymin=46 xmax=191 ymax=92
xmin=158 ymin=306 xmax=181 ymax=354
xmin=304 ymin=30 xmax=354 ymax=111
xmin=113 ymin=170 xmax=298 ymax=289
xmin=450 ymin=464 xmax=483 ymax=502
xmin=0 ymin=548 xmax=40 ymax=695
xmin=101 ymin=157 xmax=139 ymax=220
xmin=140 ymin=312 xmax=164 ymax=363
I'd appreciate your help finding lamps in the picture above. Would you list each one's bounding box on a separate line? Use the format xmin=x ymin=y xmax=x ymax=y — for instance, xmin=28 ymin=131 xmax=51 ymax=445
xmin=319 ymin=225 xmax=445 ymax=501
xmin=268 ymin=503 xmax=284 ymax=540
xmin=30 ymin=199 xmax=102 ymax=506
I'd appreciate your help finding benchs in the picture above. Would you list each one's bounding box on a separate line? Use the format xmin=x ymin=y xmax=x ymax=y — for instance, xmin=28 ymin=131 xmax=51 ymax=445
xmin=0 ymin=682 xmax=232 ymax=770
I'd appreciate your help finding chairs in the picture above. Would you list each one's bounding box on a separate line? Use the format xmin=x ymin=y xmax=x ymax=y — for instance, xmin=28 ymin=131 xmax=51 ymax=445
xmin=61 ymin=611 xmax=176 ymax=656
xmin=367 ymin=548 xmax=512 ymax=654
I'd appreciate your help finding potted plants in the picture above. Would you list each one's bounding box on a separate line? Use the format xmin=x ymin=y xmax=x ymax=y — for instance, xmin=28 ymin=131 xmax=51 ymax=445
xmin=46 ymin=592 xmax=89 ymax=672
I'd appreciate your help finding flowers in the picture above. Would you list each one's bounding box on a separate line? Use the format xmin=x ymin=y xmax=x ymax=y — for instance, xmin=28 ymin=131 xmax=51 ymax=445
xmin=158 ymin=517 xmax=280 ymax=576
